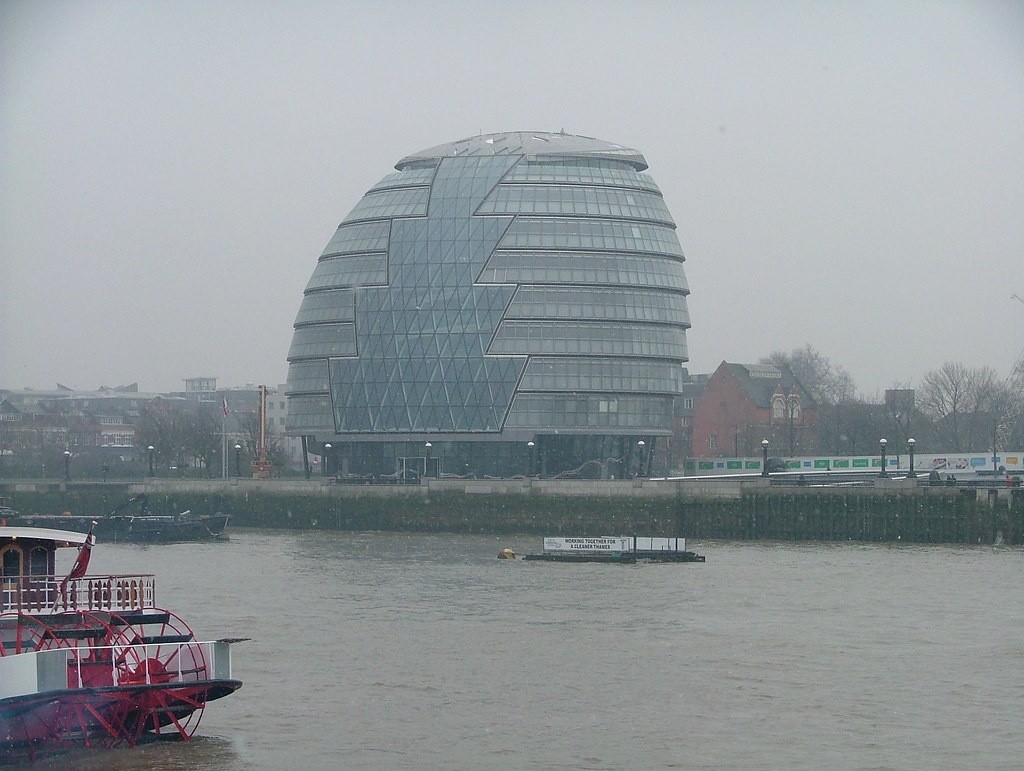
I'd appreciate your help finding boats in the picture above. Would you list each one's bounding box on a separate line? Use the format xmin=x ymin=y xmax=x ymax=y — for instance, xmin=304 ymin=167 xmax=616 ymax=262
xmin=0 ymin=506 xmax=232 ymax=543
xmin=0 ymin=523 xmax=247 ymax=766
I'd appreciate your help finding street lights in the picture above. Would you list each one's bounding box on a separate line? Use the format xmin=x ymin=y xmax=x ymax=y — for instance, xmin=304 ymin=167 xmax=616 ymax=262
xmin=324 ymin=444 xmax=333 ymax=476
xmin=907 ymin=438 xmax=918 ymax=479
xmin=762 ymin=438 xmax=770 ymax=478
xmin=63 ymin=451 xmax=71 ymax=481
xmin=877 ymin=438 xmax=889 ymax=478
xmin=232 ymin=444 xmax=242 ymax=478
xmin=424 ymin=443 xmax=432 ymax=477
xmin=638 ymin=440 xmax=646 ymax=476
xmin=527 ymin=441 xmax=536 ymax=477
xmin=148 ymin=445 xmax=155 ymax=477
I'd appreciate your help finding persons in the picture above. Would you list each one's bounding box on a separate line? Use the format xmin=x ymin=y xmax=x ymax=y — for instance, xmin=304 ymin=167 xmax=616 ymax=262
xmin=797 ymin=473 xmax=805 ymax=487
xmin=1006 ymin=475 xmax=1011 ymax=486
xmin=946 ymin=474 xmax=956 ymax=486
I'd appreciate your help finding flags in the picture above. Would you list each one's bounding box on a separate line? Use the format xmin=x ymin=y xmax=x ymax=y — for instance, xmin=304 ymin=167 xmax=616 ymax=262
xmin=61 ymin=530 xmax=93 ymax=593
xmin=223 ymin=397 xmax=228 ymax=417
